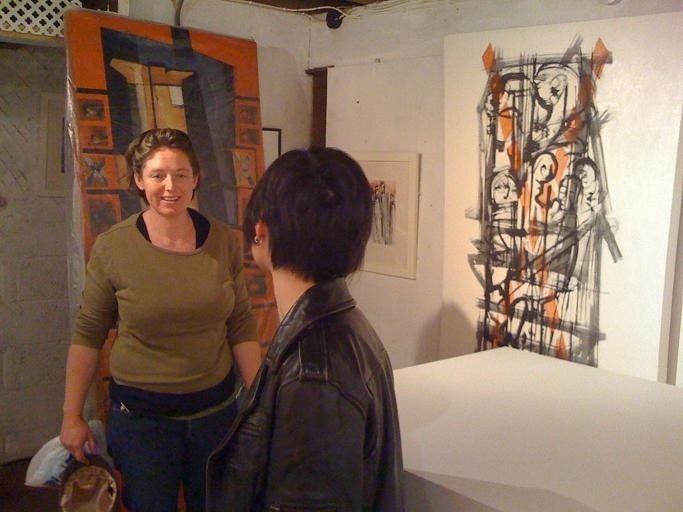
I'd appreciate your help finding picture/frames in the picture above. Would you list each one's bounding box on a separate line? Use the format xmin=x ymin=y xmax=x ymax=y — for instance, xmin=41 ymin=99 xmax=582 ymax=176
xmin=37 ymin=92 xmax=72 ymax=198
xmin=349 ymin=151 xmax=421 ymax=280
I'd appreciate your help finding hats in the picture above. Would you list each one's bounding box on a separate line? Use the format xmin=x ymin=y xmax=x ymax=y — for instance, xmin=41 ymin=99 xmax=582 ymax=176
xmin=58 ymin=454 xmax=118 ymax=512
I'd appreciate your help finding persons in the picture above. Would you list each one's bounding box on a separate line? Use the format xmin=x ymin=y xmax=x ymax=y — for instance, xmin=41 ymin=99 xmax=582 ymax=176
xmin=59 ymin=128 xmax=264 ymax=511
xmin=205 ymin=147 xmax=403 ymax=512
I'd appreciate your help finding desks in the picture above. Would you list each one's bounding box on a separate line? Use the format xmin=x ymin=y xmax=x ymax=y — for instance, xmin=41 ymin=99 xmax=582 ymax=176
xmin=392 ymin=347 xmax=683 ymax=512
